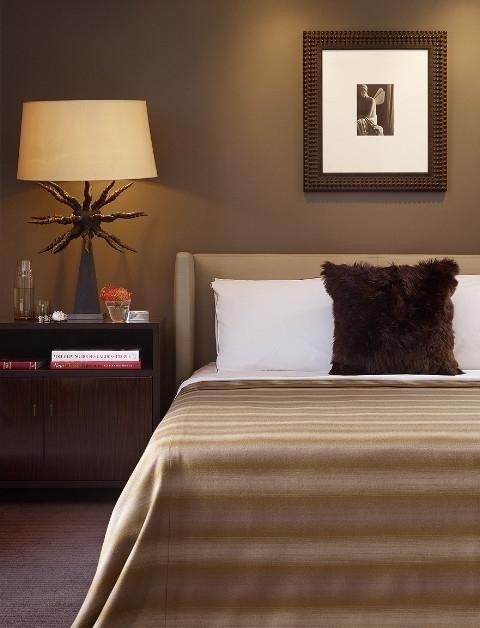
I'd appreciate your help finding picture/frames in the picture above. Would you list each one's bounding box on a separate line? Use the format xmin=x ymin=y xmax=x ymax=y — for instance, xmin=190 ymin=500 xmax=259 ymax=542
xmin=303 ymin=30 xmax=447 ymax=191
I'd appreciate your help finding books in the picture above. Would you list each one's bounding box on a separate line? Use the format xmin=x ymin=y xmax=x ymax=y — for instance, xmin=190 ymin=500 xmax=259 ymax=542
xmin=51 ymin=350 xmax=139 ymax=361
xmin=50 ymin=361 xmax=141 ymax=370
xmin=1 ymin=358 xmax=46 ymax=370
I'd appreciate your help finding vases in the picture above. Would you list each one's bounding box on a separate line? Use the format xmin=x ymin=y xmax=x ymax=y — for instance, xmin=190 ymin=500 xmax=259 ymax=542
xmin=105 ymin=302 xmax=130 ymax=322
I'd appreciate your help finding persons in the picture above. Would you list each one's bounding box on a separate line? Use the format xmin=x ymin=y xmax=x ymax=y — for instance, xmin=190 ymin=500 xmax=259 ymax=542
xmin=357 ymin=84 xmax=385 ymax=136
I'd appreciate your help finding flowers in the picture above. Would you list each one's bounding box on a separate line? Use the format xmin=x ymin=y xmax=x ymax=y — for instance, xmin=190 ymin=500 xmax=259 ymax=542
xmin=99 ymin=282 xmax=137 ymax=301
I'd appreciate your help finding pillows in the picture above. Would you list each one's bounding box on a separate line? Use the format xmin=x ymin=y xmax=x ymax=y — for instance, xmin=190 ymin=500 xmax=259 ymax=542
xmin=320 ymin=257 xmax=466 ymax=375
xmin=210 ymin=278 xmax=334 ymax=373
xmin=451 ymin=275 xmax=480 ymax=370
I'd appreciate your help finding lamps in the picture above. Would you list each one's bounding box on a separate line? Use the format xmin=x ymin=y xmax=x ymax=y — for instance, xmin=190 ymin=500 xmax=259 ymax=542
xmin=17 ymin=100 xmax=158 ymax=320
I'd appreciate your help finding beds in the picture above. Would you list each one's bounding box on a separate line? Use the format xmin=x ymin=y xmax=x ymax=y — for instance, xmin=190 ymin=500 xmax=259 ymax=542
xmin=71 ymin=252 xmax=480 ymax=628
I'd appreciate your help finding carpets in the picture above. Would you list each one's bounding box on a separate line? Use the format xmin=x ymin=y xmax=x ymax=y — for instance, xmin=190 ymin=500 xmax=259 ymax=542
xmin=0 ymin=502 xmax=113 ymax=627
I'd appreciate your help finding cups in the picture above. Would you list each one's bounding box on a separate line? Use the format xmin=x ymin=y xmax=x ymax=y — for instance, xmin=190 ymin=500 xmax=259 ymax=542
xmin=13 ymin=258 xmax=34 ymax=320
xmin=107 ymin=305 xmax=129 ymax=322
xmin=36 ymin=298 xmax=49 ymax=323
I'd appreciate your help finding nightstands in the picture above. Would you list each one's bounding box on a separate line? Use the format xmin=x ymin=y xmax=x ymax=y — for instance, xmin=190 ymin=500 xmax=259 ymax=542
xmin=0 ymin=317 xmax=167 ymax=488
